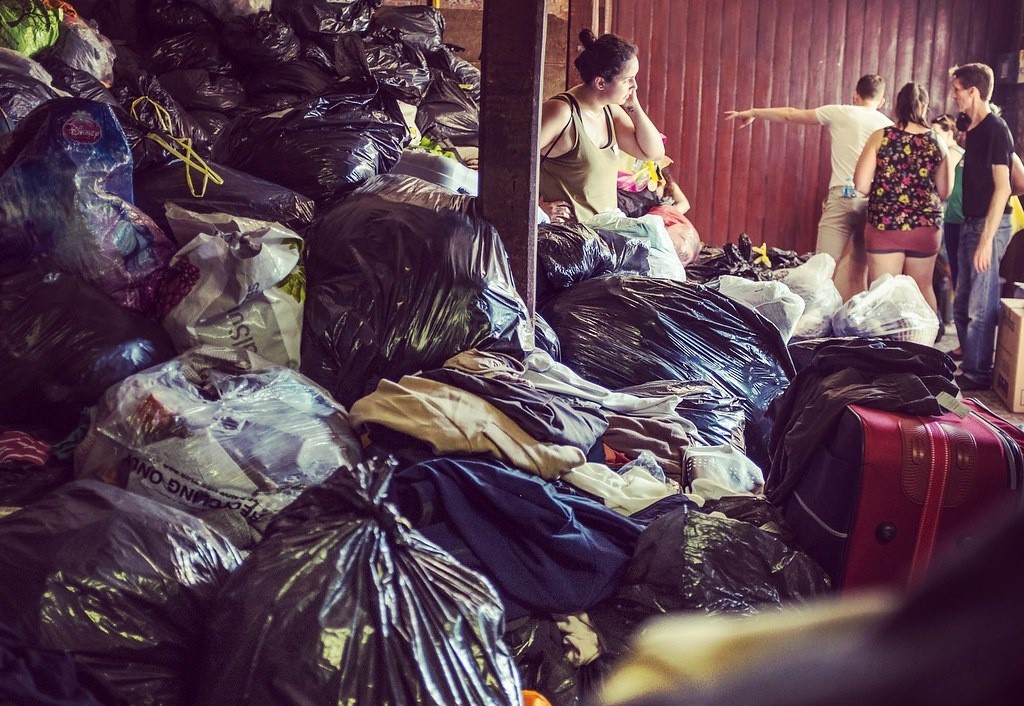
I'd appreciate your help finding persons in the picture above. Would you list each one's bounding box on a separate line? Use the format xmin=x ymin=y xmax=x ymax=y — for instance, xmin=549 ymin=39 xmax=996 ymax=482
xmin=538 ymin=28 xmax=664 ymax=226
xmin=658 ymin=155 xmax=691 ymax=215
xmin=725 ymin=62 xmax=1024 ymax=391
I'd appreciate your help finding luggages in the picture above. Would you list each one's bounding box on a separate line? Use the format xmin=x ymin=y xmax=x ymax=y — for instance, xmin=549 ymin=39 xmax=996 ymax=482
xmin=790 ymin=395 xmax=1024 ymax=596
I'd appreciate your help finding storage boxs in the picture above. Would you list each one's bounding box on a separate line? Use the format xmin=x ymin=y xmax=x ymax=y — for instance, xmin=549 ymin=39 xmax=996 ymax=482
xmin=992 ymin=298 xmax=1024 ymax=414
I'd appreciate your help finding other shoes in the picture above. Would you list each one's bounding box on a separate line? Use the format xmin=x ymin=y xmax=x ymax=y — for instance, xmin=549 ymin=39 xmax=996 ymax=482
xmin=942 ymin=320 xmax=958 ymax=334
xmin=954 ymin=371 xmax=989 ymax=390
xmin=948 ymin=347 xmax=964 ymax=361
